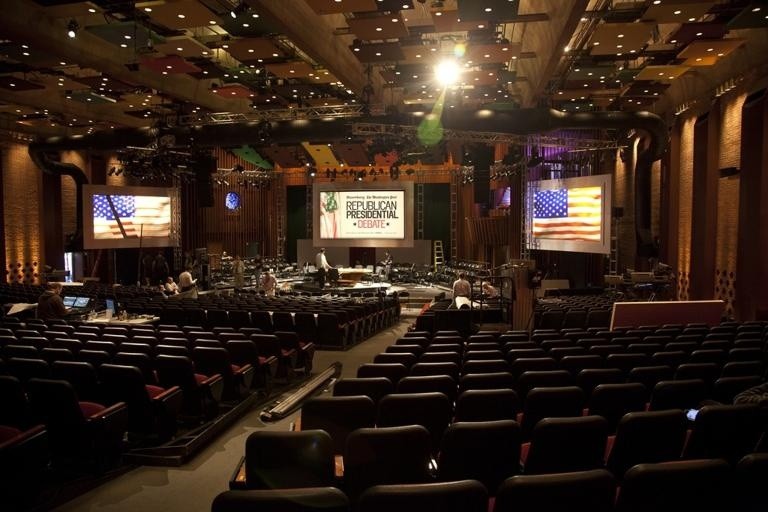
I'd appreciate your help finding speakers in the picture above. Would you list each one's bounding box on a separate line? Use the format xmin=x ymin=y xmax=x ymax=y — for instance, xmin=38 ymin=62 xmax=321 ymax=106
xmin=720 ymin=167 xmax=737 ymax=178
xmin=472 ymin=141 xmax=490 ymax=204
xmin=196 ymin=143 xmax=216 ymax=208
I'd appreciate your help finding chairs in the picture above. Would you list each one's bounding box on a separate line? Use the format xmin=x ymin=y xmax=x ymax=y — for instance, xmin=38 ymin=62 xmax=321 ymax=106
xmin=0 ymin=264 xmax=768 ymax=512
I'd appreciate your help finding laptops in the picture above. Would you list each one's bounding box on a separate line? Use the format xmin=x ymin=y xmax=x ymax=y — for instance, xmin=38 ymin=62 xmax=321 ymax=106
xmin=62 ymin=296 xmax=77 ymax=310
xmin=65 ymin=297 xmax=92 ymax=312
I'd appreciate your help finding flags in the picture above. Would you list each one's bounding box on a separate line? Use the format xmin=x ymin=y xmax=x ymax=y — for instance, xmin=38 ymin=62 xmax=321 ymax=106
xmin=93 ymin=194 xmax=171 ymax=239
xmin=532 ymin=186 xmax=601 ymax=241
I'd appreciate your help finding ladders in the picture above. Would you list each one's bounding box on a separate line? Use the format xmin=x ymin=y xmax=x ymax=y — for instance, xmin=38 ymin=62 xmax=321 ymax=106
xmin=434 ymin=241 xmax=445 ymax=273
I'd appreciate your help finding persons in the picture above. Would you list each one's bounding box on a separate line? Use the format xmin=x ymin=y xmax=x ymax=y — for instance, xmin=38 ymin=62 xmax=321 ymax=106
xmin=37 ymin=283 xmax=73 ymax=319
xmin=140 ymin=252 xmax=198 ymax=295
xmin=315 ymin=248 xmax=332 ymax=272
xmin=222 ymin=252 xmax=277 ymax=291
xmin=482 ymin=281 xmax=496 ymax=294
xmin=380 ymin=249 xmax=393 ymax=268
xmin=452 ymin=272 xmax=471 ymax=298
xmin=648 ymin=250 xmax=674 ymax=299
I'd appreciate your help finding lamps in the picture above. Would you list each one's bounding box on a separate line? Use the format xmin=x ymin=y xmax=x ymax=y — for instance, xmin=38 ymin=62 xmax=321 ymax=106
xmin=105 ymin=150 xmax=627 ymax=186
